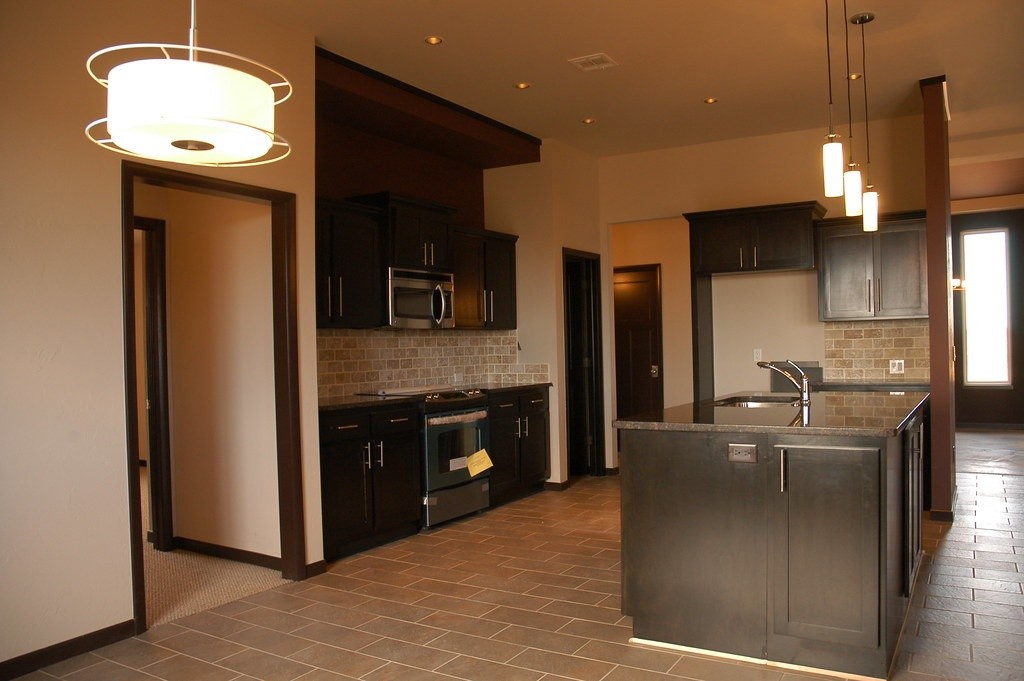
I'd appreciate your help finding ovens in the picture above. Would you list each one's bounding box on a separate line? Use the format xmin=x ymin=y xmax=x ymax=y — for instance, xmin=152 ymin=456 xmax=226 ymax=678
xmin=420 ymin=407 xmax=490 ymax=526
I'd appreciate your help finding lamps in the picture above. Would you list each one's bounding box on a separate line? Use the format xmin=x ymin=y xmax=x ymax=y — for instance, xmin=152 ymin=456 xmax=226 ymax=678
xmin=850 ymin=12 xmax=878 ymax=231
xmin=843 ymin=0 xmax=862 ymax=217
xmin=822 ymin=0 xmax=844 ymax=198
xmin=85 ymin=0 xmax=293 ymax=168
xmin=953 ymin=274 xmax=966 ymax=291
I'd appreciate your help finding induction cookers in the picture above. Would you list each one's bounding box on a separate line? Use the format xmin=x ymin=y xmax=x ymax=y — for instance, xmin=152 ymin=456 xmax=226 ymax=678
xmin=355 ymin=383 xmax=487 ymax=408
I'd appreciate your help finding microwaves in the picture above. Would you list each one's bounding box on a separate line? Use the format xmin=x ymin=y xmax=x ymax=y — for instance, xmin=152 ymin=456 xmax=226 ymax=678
xmin=380 ymin=265 xmax=456 ymax=332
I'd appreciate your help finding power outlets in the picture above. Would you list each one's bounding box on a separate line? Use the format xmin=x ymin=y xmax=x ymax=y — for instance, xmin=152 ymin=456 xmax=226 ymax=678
xmin=729 ymin=443 xmax=759 ymax=464
xmin=455 ymin=373 xmax=464 ymax=381
xmin=753 ymin=349 xmax=761 ymax=361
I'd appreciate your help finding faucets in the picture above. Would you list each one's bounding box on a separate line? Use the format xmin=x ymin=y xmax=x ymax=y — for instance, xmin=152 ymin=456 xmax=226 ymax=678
xmin=756 ymin=358 xmax=810 ymax=402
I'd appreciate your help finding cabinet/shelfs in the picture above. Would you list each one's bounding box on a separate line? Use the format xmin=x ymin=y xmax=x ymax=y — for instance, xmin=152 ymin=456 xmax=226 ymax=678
xmin=387 ymin=204 xmax=451 ymax=270
xmin=315 ymin=206 xmax=384 ymax=330
xmin=449 ymin=226 xmax=519 ymax=331
xmin=818 ymin=218 xmax=928 ymax=322
xmin=700 ymin=203 xmax=828 ymax=276
xmin=487 ymin=389 xmax=550 ymax=501
xmin=318 ymin=403 xmax=421 ymax=553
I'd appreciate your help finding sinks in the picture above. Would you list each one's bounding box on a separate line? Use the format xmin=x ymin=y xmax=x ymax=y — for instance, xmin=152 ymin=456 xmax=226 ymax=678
xmin=714 ymin=394 xmax=802 ymax=407
